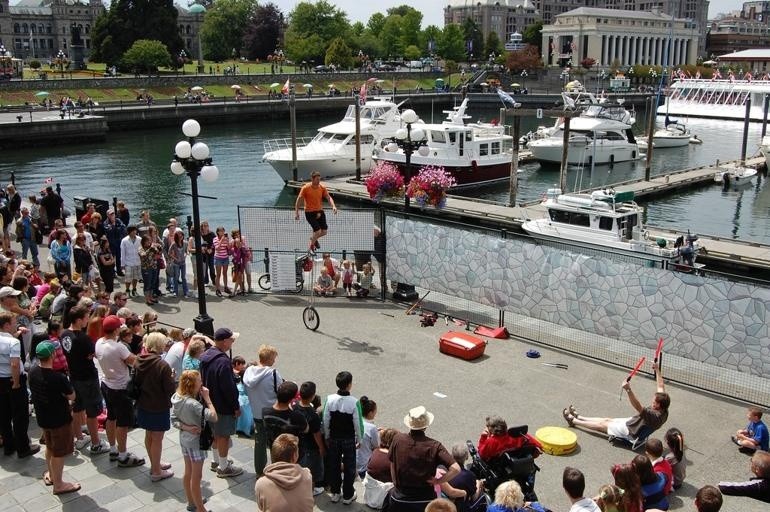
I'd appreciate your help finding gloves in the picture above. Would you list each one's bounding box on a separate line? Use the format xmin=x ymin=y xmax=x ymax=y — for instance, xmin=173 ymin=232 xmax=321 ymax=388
xmin=261 ymin=74 xmax=408 ymax=189
xmin=374 ymin=90 xmax=527 ymax=184
xmin=517 ymin=123 xmax=706 ymax=274
xmin=528 ymin=79 xmax=704 ymax=165
xmin=756 ymin=142 xmax=770 ymax=175
xmin=713 ymin=166 xmax=759 ymax=186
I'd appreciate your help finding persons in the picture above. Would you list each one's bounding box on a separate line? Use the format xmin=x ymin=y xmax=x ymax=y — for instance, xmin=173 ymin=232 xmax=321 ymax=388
xmin=563 ymin=363 xmax=671 ymax=444
xmin=269 ymin=89 xmax=277 ymax=100
xmin=137 ymin=93 xmax=153 ymax=105
xmin=58 ymin=96 xmax=83 ymax=108
xmin=673 ymin=236 xmax=684 ymax=248
xmin=106 ymin=64 xmax=117 ymax=77
xmin=184 ymin=87 xmax=210 ymax=103
xmin=514 ymin=88 xmax=527 ymax=95
xmin=732 ymin=406 xmax=768 ymax=454
xmin=226 ymin=65 xmax=240 ymax=75
xmin=718 ymin=450 xmax=769 ymax=503
xmin=441 ymin=82 xmax=449 ymax=93
xmin=0 ymin=187 xmax=552 ymax=512
xmin=294 ymin=172 xmax=337 ymax=255
xmin=307 ymin=88 xmax=313 ymax=98
xmin=562 ymin=466 xmax=601 ymax=510
xmin=235 ymin=89 xmax=241 ymax=103
xmin=415 ymin=85 xmax=420 ymax=94
xmin=594 ymin=428 xmax=687 ymax=510
xmin=689 ymin=485 xmax=723 ymax=510
xmin=329 ymin=85 xmax=383 ymax=95
xmin=43 ymin=98 xmax=53 ymax=107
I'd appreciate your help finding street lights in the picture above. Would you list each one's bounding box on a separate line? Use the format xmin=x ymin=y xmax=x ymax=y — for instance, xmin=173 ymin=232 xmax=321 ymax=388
xmin=200 ymin=406 xmax=215 ymax=450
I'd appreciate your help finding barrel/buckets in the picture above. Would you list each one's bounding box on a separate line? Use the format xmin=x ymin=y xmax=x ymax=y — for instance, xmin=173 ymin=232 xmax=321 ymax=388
xmin=42 ymin=470 xmax=81 ymax=495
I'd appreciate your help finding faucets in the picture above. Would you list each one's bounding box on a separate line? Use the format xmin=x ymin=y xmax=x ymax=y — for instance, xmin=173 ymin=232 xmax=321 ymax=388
xmin=563 ymin=404 xmax=582 ymax=425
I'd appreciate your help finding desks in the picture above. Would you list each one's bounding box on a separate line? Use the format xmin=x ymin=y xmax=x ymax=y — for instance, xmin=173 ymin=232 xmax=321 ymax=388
xmin=561 ymin=68 xmax=569 ymax=88
xmin=358 ymin=49 xmax=364 ymax=72
xmin=520 ymin=69 xmax=529 ymax=90
xmin=699 ymin=55 xmax=704 ymax=64
xmin=231 ymin=47 xmax=237 ymax=74
xmin=0 ymin=44 xmax=8 ymax=81
xmin=57 ymin=48 xmax=68 ymax=78
xmin=651 ymin=69 xmax=658 ymax=85
xmin=394 ymin=102 xmax=431 ymax=210
xmin=627 ymin=65 xmax=634 ymax=76
xmin=180 ymin=49 xmax=188 ymax=75
xmin=168 ymin=116 xmax=218 ymax=339
xmin=599 ymin=67 xmax=607 ymax=89
xmin=710 ymin=52 xmax=715 ymax=61
xmin=646 ymin=66 xmax=654 ymax=82
xmin=566 ymin=57 xmax=574 ymax=68
xmin=278 ymin=48 xmax=284 ymax=74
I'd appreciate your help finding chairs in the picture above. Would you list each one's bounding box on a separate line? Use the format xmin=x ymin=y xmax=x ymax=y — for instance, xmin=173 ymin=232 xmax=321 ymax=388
xmin=186 ymin=498 xmax=212 ymax=512
xmin=316 ymin=285 xmax=353 ymax=298
xmin=312 ymin=487 xmax=325 ymax=496
xmin=89 ymin=439 xmax=112 ymax=455
xmin=109 ymin=450 xmax=146 ymax=468
xmin=343 ymin=491 xmax=358 ymax=505
xmin=75 ymin=433 xmax=91 ymax=450
xmin=187 ymin=306 xmax=190 ymax=309
xmin=209 ymin=459 xmax=245 ymax=478
xmin=0 ymin=435 xmax=48 ymax=460
xmin=308 ymin=239 xmax=321 ymax=256
xmin=148 ymin=461 xmax=175 ymax=482
xmin=731 ymin=435 xmax=743 ymax=449
xmin=124 ymin=277 xmax=254 ymax=309
xmin=330 ymin=491 xmax=342 ymax=503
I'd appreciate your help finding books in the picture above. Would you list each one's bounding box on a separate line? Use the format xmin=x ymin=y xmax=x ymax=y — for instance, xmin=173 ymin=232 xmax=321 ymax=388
xmin=437 ymin=328 xmax=486 ymax=361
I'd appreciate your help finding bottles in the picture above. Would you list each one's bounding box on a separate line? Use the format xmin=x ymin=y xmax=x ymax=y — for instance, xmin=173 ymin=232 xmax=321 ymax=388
xmin=0 ymin=254 xmax=12 ymax=267
xmin=214 ymin=328 xmax=241 ymax=341
xmin=106 ymin=209 xmax=116 ymax=217
xmin=102 ymin=315 xmax=135 ymax=343
xmin=402 ymin=404 xmax=436 ymax=431
xmin=35 ymin=339 xmax=62 ymax=360
xmin=182 ymin=328 xmax=198 ymax=339
xmin=0 ymin=286 xmax=23 ymax=299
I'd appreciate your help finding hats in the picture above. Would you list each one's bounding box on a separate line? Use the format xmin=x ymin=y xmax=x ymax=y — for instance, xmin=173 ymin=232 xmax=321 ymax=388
xmin=312 ymin=63 xmax=332 ymax=72
xmin=366 ymin=57 xmax=435 ymax=72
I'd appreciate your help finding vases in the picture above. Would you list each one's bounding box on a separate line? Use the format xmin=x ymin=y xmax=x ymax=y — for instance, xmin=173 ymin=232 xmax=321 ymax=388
xmin=362 ymin=161 xmax=407 ymax=203
xmin=400 ymin=163 xmax=458 ymax=209
